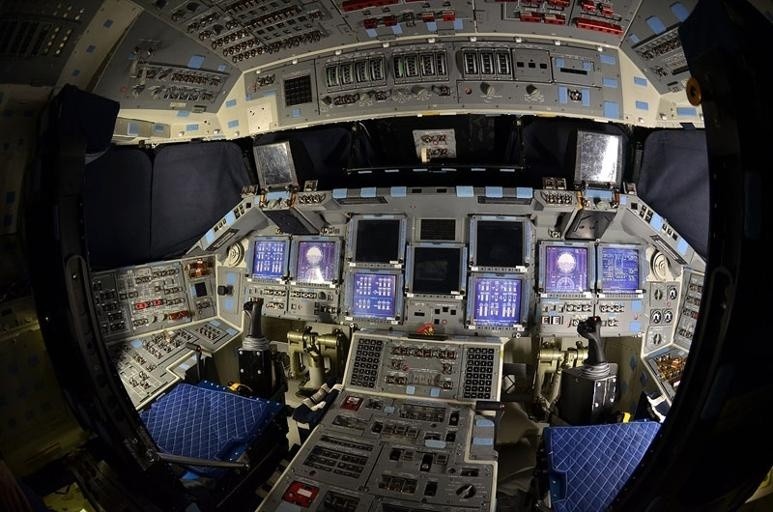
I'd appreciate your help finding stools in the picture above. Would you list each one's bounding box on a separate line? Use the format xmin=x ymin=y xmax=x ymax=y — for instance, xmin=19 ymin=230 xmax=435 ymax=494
xmin=525 ymin=419 xmax=662 ymax=512
xmin=139 ymin=380 xmax=290 ymax=512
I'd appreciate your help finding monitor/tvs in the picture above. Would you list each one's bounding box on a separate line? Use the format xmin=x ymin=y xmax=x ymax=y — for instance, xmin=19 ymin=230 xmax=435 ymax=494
xmin=249 ymin=236 xmax=290 ymax=281
xmin=344 ymin=267 xmax=404 ymax=324
xmin=414 ymin=216 xmax=464 ymax=243
xmin=288 ymin=235 xmax=342 ymax=285
xmin=595 ymin=242 xmax=647 ymax=294
xmin=468 ymin=215 xmax=531 ymax=272
xmin=538 ymin=239 xmax=596 ymax=292
xmin=466 ymin=272 xmax=531 ymax=326
xmin=404 ymin=243 xmax=468 ymax=301
xmin=348 ymin=216 xmax=407 ymax=266
